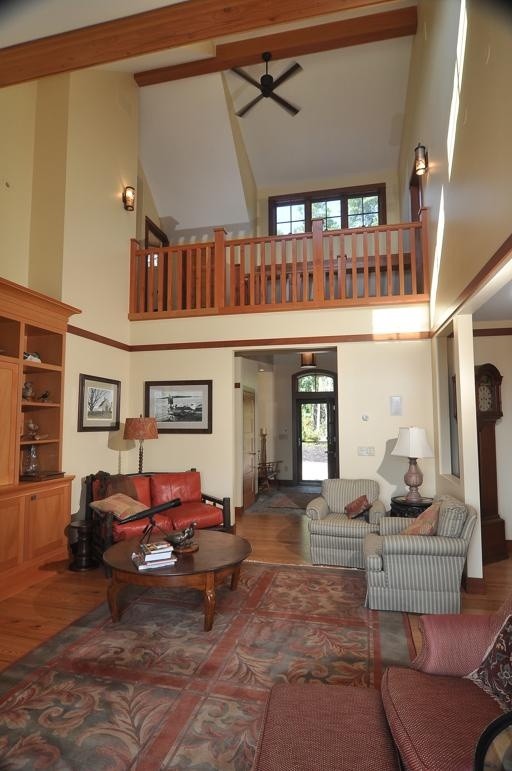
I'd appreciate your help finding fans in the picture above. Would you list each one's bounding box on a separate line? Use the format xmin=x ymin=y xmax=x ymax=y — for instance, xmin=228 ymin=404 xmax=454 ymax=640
xmin=225 ymin=48 xmax=303 ymax=120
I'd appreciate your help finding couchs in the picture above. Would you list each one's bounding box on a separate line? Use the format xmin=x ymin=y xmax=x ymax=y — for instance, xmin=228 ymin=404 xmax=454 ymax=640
xmin=307 ymin=478 xmax=384 ymax=571
xmin=382 ymin=586 xmax=512 ymax=770
xmin=365 ymin=492 xmax=464 ymax=616
xmin=84 ymin=468 xmax=233 ymax=580
xmin=251 ymin=684 xmax=401 ymax=769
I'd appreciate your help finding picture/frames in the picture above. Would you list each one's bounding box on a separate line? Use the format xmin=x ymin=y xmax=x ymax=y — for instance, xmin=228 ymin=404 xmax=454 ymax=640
xmin=144 ymin=379 xmax=213 ymax=434
xmin=77 ymin=372 xmax=121 ymax=435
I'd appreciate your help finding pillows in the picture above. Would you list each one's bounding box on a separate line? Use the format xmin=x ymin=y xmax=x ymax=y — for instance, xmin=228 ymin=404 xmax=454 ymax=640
xmin=398 ymin=502 xmax=438 ymax=537
xmin=88 ymin=493 xmax=150 ymax=521
xmin=343 ymin=496 xmax=370 ymax=518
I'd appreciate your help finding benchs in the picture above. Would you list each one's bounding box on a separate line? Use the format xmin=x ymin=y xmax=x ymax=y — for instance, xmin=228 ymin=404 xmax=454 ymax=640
xmin=256 ymin=462 xmax=283 ymax=496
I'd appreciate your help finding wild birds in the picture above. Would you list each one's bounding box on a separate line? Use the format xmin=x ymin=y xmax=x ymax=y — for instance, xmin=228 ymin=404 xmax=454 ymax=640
xmin=182 ymin=521 xmax=198 ymax=539
xmin=163 ymin=528 xmax=191 ymax=548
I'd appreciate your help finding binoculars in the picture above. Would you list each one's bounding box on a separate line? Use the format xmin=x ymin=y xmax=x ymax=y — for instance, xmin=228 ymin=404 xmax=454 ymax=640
xmin=119 ymin=498 xmax=181 ymax=525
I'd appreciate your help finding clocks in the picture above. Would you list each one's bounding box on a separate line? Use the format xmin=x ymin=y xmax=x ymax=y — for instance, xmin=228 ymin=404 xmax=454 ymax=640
xmin=450 ymin=363 xmax=512 ymax=566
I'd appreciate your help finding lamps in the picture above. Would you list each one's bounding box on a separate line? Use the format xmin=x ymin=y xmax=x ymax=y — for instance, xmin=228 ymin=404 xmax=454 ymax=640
xmin=124 ymin=415 xmax=158 ymax=473
xmin=414 ymin=143 xmax=428 ymax=175
xmin=123 ymin=185 xmax=136 ymax=211
xmin=390 ymin=426 xmax=437 ymax=501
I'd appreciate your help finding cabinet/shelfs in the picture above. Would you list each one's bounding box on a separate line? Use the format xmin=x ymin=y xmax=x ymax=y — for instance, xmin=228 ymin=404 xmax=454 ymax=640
xmin=0 ymin=274 xmax=82 ymax=484
xmin=0 ymin=474 xmax=74 ymax=582
xmin=390 ymin=497 xmax=433 ymax=518
xmin=69 ymin=518 xmax=99 ymax=573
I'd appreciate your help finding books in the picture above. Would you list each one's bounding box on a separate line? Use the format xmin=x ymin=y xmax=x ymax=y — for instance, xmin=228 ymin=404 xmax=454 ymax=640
xmin=131 ymin=541 xmax=178 ymax=570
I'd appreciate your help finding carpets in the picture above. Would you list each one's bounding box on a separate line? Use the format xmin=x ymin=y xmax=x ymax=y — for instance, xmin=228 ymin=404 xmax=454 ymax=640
xmin=0 ymin=558 xmax=418 ymax=771
xmin=268 ymin=493 xmax=320 ymax=509
